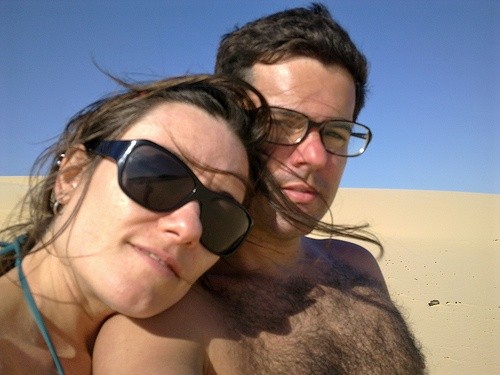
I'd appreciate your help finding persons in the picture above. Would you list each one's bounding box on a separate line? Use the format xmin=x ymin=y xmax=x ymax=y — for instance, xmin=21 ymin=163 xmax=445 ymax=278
xmin=89 ymin=0 xmax=429 ymax=375
xmin=0 ymin=58 xmax=386 ymax=375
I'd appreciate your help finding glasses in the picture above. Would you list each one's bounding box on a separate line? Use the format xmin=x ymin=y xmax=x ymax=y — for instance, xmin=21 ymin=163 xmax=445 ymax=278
xmin=254 ymin=106 xmax=372 ymax=157
xmin=82 ymin=139 xmax=254 ymax=257
